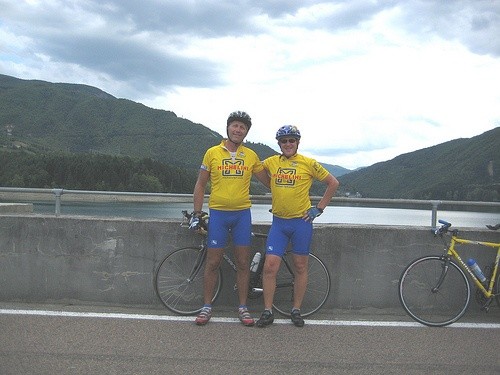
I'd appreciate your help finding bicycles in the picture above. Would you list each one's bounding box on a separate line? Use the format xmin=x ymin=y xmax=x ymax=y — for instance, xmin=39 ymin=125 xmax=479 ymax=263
xmin=397 ymin=219 xmax=500 ymax=329
xmin=153 ymin=210 xmax=330 ymax=318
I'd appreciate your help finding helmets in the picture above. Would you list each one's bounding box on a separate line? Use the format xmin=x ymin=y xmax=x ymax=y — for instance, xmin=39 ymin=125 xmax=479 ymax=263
xmin=227 ymin=110 xmax=252 ymax=127
xmin=276 ymin=125 xmax=301 ymax=139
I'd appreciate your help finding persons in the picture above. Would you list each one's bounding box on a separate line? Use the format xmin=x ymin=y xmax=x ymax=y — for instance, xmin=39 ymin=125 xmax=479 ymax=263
xmin=254 ymin=126 xmax=339 ymax=327
xmin=188 ymin=111 xmax=272 ymax=325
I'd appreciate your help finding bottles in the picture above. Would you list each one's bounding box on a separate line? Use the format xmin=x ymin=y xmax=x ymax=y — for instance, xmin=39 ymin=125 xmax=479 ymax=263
xmin=250 ymin=252 xmax=261 ymax=272
xmin=467 ymin=259 xmax=486 ymax=282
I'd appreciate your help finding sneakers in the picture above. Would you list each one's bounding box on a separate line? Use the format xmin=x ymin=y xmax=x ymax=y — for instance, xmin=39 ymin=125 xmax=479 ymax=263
xmin=291 ymin=310 xmax=305 ymax=327
xmin=195 ymin=306 xmax=212 ymax=324
xmin=239 ymin=308 xmax=254 ymax=325
xmin=255 ymin=310 xmax=275 ymax=326
